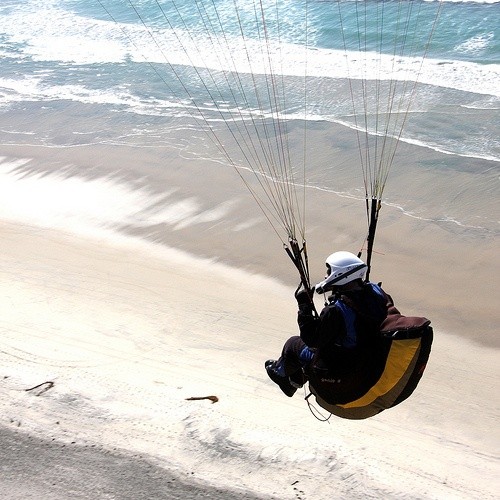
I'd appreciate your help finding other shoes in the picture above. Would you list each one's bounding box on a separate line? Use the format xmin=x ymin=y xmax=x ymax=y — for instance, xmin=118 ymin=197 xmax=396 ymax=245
xmin=265 ymin=359 xmax=277 ymax=369
xmin=266 ymin=366 xmax=298 ymax=397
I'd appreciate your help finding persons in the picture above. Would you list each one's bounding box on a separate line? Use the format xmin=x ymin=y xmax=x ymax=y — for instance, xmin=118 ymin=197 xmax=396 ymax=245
xmin=265 ymin=251 xmax=394 ymax=397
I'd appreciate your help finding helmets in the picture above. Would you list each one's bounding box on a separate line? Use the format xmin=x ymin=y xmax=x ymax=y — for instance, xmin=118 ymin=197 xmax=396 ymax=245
xmin=315 ymin=251 xmax=368 ymax=294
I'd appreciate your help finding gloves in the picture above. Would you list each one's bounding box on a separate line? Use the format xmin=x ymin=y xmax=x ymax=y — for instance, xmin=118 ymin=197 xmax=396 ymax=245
xmin=295 ymin=286 xmax=315 ymax=305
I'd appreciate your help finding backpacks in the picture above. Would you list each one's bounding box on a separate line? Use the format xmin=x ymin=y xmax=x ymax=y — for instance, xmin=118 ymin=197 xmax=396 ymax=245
xmin=308 ymin=282 xmax=433 ymax=420
